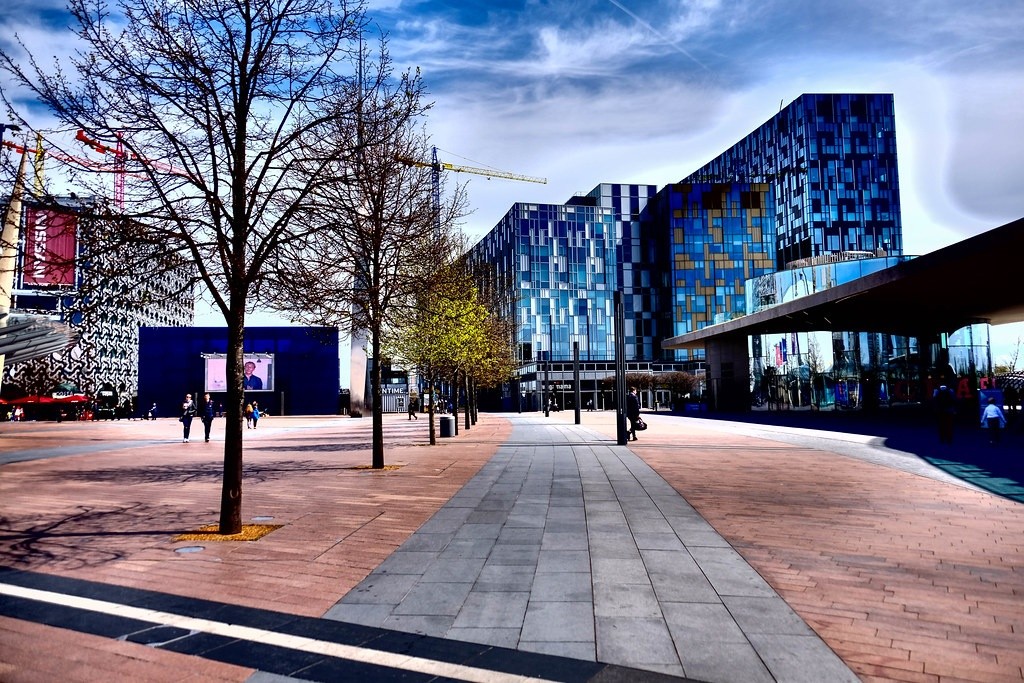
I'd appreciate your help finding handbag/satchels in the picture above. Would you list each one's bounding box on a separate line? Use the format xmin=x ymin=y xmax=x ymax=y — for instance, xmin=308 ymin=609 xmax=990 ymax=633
xmin=636 ymin=418 xmax=647 ymax=430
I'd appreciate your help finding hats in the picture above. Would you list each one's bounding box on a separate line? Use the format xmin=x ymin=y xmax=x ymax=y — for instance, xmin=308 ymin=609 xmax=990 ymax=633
xmin=153 ymin=403 xmax=156 ymax=405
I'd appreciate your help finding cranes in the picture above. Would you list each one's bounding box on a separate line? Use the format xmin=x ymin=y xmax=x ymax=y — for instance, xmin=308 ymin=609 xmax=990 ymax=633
xmin=389 ymin=144 xmax=547 ymax=245
xmin=75 ymin=127 xmax=205 ymax=212
xmin=2 ymin=132 xmax=150 ymax=196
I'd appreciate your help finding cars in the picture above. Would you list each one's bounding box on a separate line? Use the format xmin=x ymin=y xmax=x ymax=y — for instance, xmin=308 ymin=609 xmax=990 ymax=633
xmin=96 ymin=402 xmax=129 ymax=421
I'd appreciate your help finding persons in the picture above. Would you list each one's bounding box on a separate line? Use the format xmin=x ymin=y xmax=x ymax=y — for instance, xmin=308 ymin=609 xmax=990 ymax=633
xmin=73 ymin=405 xmax=85 ymax=421
xmin=979 ymin=395 xmax=1008 ymax=445
xmin=178 ymin=394 xmax=197 ymax=443
xmin=934 ymin=376 xmax=958 ymax=444
xmin=244 ymin=401 xmax=260 ymax=429
xmin=408 ymin=399 xmax=418 ymax=421
xmin=219 ymin=403 xmax=222 ymax=417
xmin=244 ymin=361 xmax=263 ymax=390
xmin=200 ymin=393 xmax=217 ymax=442
xmin=626 ymin=386 xmax=640 ymax=441
xmin=148 ymin=403 xmax=157 ymax=420
xmin=587 ymin=398 xmax=593 ymax=412
xmin=11 ymin=406 xmax=21 ymax=423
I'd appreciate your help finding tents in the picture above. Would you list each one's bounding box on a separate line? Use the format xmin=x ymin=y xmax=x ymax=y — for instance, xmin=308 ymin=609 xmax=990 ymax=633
xmin=0 ymin=395 xmax=87 ymax=405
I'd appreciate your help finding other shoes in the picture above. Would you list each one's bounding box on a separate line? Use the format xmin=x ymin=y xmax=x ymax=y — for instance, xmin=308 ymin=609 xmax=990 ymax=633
xmin=248 ymin=426 xmax=251 ymax=429
xmin=254 ymin=427 xmax=256 ymax=429
xmin=205 ymin=439 xmax=209 ymax=442
xmin=633 ymin=437 xmax=638 ymax=441
xmin=415 ymin=417 xmax=417 ymax=420
xmin=183 ymin=438 xmax=189 ymax=442
xmin=627 ymin=431 xmax=631 ymax=440
xmin=408 ymin=419 xmax=411 ymax=420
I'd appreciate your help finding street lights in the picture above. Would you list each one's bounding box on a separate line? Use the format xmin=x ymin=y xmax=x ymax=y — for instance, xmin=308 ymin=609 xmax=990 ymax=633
xmin=0 ymin=123 xmax=22 ymax=150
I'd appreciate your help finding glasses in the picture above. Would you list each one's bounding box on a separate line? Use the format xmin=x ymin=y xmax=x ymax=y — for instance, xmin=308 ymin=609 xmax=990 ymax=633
xmin=187 ymin=396 xmax=191 ymax=398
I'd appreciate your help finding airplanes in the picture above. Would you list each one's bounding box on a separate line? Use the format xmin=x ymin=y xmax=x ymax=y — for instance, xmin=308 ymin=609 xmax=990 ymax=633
xmin=0 ymin=383 xmax=89 ymax=423
